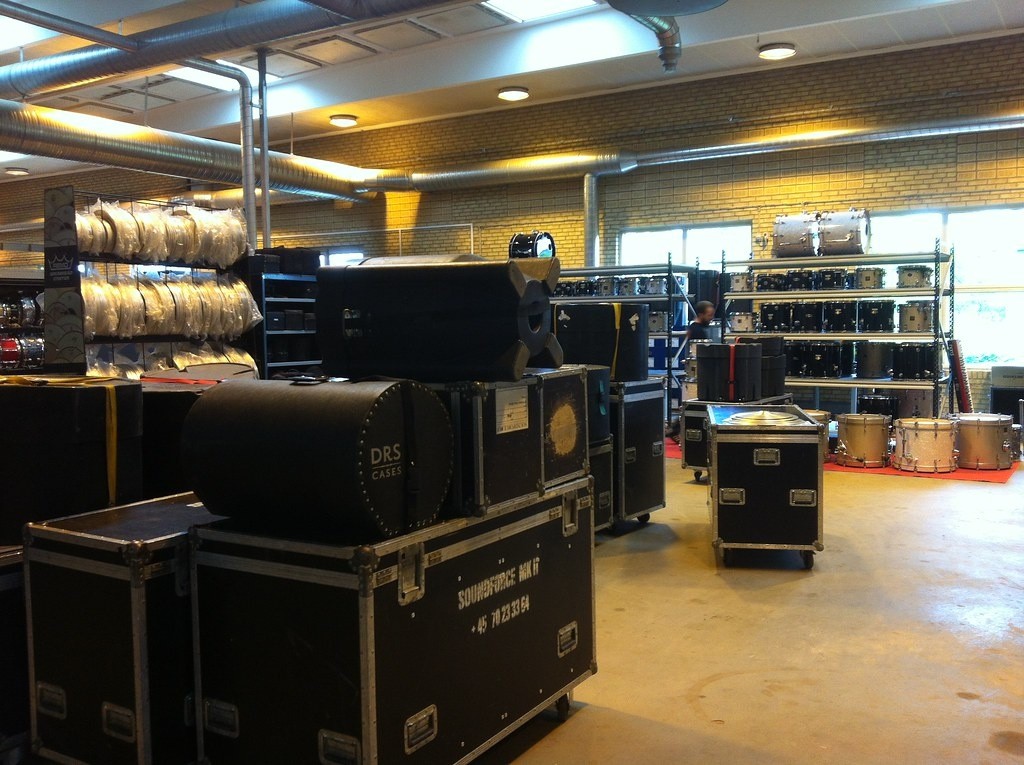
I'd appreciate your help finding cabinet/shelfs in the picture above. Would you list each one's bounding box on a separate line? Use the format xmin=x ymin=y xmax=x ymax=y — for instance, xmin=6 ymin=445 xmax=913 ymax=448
xmin=549 ymin=252 xmax=703 ymax=450
xmin=678 ymin=393 xmax=794 ymax=483
xmin=610 ymin=377 xmax=668 ymax=534
xmin=20 ymin=492 xmax=229 ymax=764
xmin=41 ymin=185 xmax=258 ymax=377
xmin=0 ymin=267 xmax=45 ymax=373
xmin=425 ymin=382 xmax=543 ymax=519
xmin=523 ymin=367 xmax=591 ymax=487
xmin=190 ymin=474 xmax=597 ymax=764
xmin=720 ymin=235 xmax=955 ymax=419
xmin=702 ymin=404 xmax=827 ymax=579
xmin=562 ymin=363 xmax=615 ymax=539
xmin=254 ymin=272 xmax=322 ymax=379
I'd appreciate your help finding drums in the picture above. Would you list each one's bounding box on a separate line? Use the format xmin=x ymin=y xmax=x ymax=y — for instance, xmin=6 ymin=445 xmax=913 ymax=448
xmin=509 ymin=207 xmax=1022 ymax=473
xmin=0 ymin=284 xmax=45 ymax=368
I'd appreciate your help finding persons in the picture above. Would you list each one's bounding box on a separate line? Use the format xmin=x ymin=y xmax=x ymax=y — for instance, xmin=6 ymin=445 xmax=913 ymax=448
xmin=688 ymin=301 xmax=716 ymax=339
